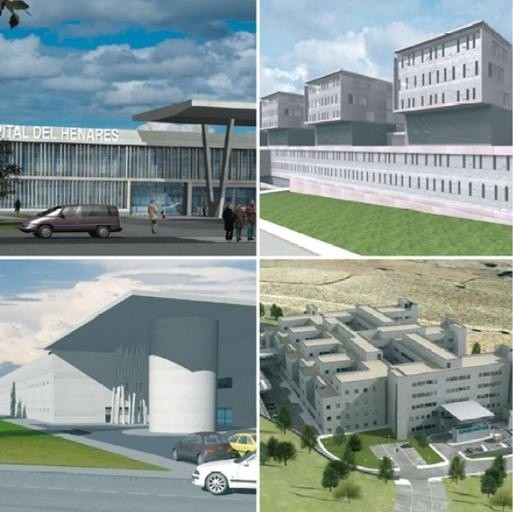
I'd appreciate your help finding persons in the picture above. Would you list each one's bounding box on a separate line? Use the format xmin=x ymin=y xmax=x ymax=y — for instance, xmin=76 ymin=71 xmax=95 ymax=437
xmin=222 ymin=203 xmax=234 ymax=241
xmin=244 ymin=199 xmax=256 ymax=240
xmin=14 ymin=198 xmax=20 ymax=214
xmin=147 ymin=198 xmax=159 ymax=233
xmin=233 ymin=202 xmax=245 ymax=243
